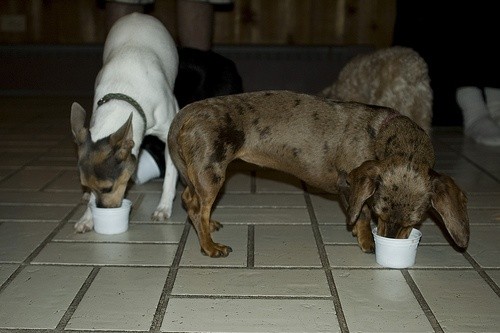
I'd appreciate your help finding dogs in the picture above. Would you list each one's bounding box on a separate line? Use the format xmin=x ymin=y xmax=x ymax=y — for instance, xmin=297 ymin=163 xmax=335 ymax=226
xmin=321 ymin=46 xmax=433 ymax=138
xmin=69 ymin=12 xmax=182 ymax=236
xmin=167 ymin=90 xmax=470 ymax=260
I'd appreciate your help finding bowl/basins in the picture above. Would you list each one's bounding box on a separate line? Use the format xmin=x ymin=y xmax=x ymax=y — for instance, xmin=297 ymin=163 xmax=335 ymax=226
xmin=130 ymin=149 xmax=160 ymax=185
xmin=371 ymin=226 xmax=423 ymax=268
xmin=89 ymin=199 xmax=132 ymax=235
